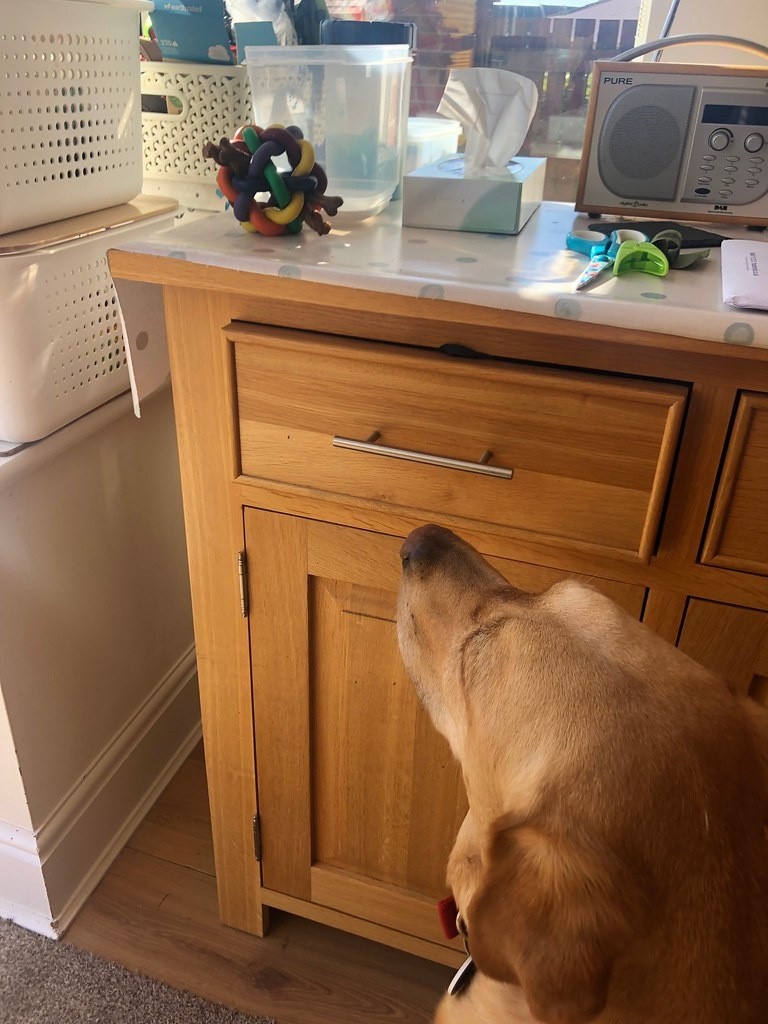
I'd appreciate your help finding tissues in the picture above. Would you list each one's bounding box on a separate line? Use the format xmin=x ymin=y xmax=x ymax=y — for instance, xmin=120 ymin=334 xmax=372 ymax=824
xmin=397 ymin=64 xmax=551 ymax=237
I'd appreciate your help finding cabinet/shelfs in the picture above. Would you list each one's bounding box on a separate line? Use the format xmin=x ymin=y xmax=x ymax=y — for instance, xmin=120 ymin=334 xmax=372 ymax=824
xmin=108 ymin=198 xmax=768 ymax=972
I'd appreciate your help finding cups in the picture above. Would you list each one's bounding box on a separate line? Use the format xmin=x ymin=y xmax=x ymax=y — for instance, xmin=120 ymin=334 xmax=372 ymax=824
xmin=490 ymin=36 xmax=547 ymax=154
xmin=319 ymin=20 xmax=418 ymax=202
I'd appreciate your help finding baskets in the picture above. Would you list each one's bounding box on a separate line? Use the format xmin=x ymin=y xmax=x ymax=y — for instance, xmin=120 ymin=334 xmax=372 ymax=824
xmin=139 ymin=62 xmax=314 ymax=211
xmin=0 ymin=205 xmax=186 ymax=443
xmin=0 ymin=0 xmax=155 ymax=237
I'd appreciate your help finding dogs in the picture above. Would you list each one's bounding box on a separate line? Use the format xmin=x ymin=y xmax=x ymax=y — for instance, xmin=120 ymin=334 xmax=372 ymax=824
xmin=387 ymin=523 xmax=768 ymax=1024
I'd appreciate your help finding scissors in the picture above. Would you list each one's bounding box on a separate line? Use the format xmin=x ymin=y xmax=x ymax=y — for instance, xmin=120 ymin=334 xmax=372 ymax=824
xmin=566 ymin=228 xmax=651 ymax=292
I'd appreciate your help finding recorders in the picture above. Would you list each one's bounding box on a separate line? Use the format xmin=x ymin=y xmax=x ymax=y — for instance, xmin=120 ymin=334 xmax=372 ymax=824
xmin=575 ymin=0 xmax=768 ymax=232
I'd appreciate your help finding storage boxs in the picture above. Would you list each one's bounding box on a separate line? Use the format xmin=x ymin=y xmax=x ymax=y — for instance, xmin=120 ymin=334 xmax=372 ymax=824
xmin=0 ymin=194 xmax=186 ymax=442
xmin=141 ymin=62 xmax=313 ymax=213
xmin=0 ymin=0 xmax=155 ymax=237
xmin=245 ymin=45 xmax=462 ymax=228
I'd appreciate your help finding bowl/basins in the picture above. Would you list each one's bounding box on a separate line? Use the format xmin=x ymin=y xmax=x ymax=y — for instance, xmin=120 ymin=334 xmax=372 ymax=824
xmin=244 ymin=45 xmax=412 ymax=224
